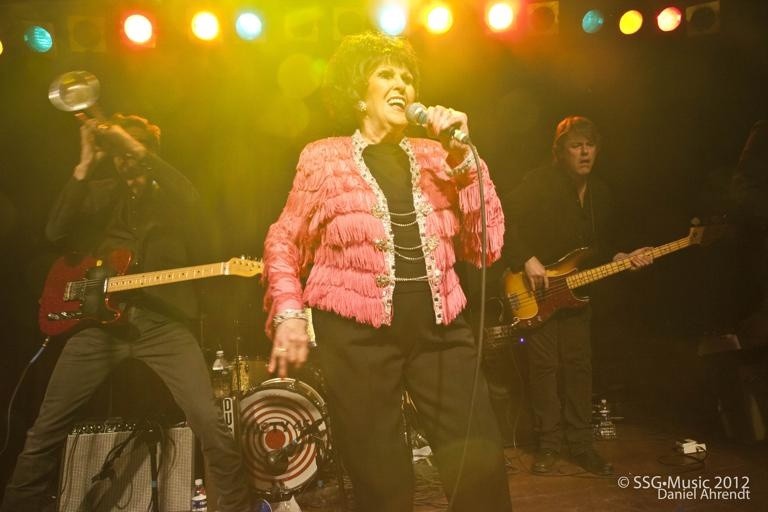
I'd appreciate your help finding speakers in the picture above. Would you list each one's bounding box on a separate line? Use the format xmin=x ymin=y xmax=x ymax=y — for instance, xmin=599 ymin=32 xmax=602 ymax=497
xmin=480 ymin=329 xmax=541 ymax=450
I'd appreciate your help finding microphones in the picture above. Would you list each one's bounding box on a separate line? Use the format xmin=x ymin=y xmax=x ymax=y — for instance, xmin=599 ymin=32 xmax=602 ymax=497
xmin=405 ymin=102 xmax=468 ymax=144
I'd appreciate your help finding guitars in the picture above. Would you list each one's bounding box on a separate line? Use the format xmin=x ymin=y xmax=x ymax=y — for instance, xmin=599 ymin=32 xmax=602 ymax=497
xmin=502 ymin=213 xmax=738 ymax=330
xmin=38 ymin=246 xmax=265 ymax=338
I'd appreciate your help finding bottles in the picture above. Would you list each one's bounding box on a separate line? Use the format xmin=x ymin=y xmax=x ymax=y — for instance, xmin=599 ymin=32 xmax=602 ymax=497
xmin=191 ymin=477 xmax=208 ymax=512
xmin=210 ymin=348 xmax=233 ymax=400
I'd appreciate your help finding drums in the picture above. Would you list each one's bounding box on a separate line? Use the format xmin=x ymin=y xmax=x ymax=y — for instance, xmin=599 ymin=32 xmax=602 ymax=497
xmin=232 ymin=355 xmax=274 ymax=394
xmin=238 ymin=378 xmax=332 ymax=502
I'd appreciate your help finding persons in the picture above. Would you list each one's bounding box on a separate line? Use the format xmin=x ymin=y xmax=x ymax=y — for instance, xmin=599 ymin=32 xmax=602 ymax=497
xmin=258 ymin=31 xmax=514 ymax=510
xmin=1 ymin=113 xmax=263 ymax=512
xmin=495 ymin=117 xmax=655 ymax=475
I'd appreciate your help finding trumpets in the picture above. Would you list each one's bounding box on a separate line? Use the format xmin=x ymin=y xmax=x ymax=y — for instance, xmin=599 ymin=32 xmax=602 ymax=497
xmin=48 ymin=70 xmax=128 ymax=161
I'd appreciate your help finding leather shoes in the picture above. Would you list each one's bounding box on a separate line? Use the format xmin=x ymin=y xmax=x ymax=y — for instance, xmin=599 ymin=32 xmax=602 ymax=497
xmin=531 ymin=451 xmax=559 ymax=476
xmin=576 ymin=448 xmax=612 ymax=476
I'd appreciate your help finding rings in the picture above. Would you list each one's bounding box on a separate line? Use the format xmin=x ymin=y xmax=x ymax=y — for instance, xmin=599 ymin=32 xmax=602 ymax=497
xmin=277 ymin=348 xmax=288 ymax=353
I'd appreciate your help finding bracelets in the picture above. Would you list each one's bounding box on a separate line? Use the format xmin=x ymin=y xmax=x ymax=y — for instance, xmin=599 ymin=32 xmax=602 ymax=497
xmin=271 ymin=310 xmax=307 ymax=329
xmin=442 ymin=145 xmax=476 ymax=178
